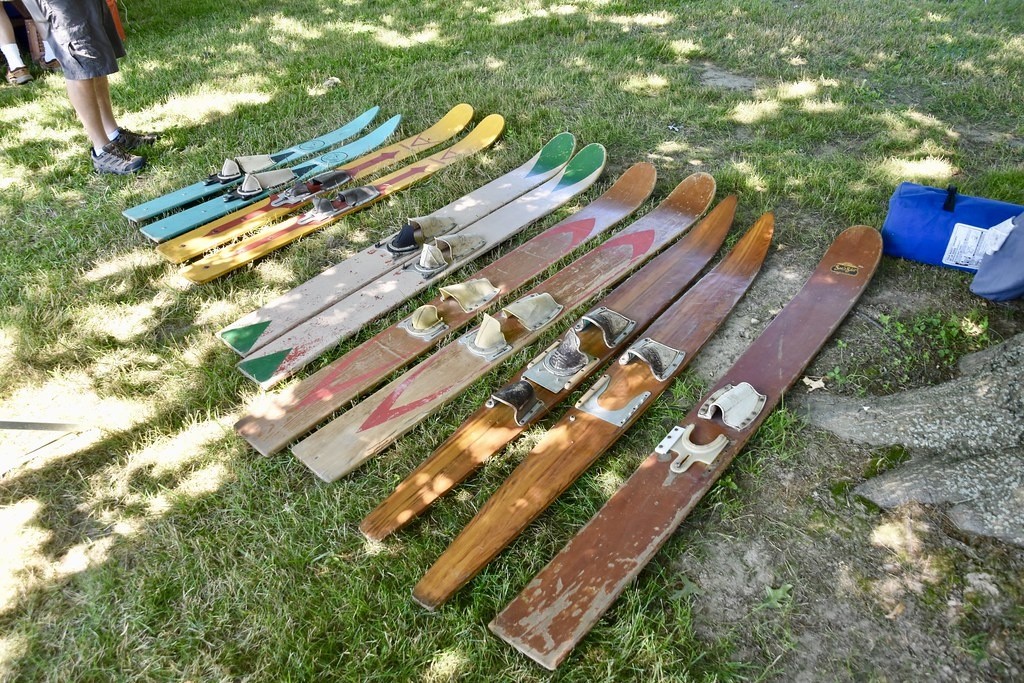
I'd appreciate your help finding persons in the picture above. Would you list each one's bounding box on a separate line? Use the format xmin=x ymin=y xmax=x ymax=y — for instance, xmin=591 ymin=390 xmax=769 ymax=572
xmin=0 ymin=0 xmax=60 ymax=85
xmin=22 ymin=0 xmax=159 ymax=173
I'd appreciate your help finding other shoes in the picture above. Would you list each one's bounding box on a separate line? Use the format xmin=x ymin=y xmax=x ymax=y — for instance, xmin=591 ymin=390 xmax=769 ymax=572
xmin=5 ymin=65 xmax=34 ymax=84
xmin=39 ymin=54 xmax=62 ymax=73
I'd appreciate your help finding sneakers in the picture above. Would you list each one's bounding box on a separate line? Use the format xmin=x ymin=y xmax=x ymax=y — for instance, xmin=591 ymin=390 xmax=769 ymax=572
xmin=91 ymin=142 xmax=144 ymax=175
xmin=110 ymin=126 xmax=161 ymax=152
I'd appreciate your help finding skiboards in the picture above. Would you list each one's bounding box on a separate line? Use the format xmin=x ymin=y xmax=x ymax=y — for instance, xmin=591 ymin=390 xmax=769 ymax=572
xmin=117 ymin=99 xmax=885 ymax=672
